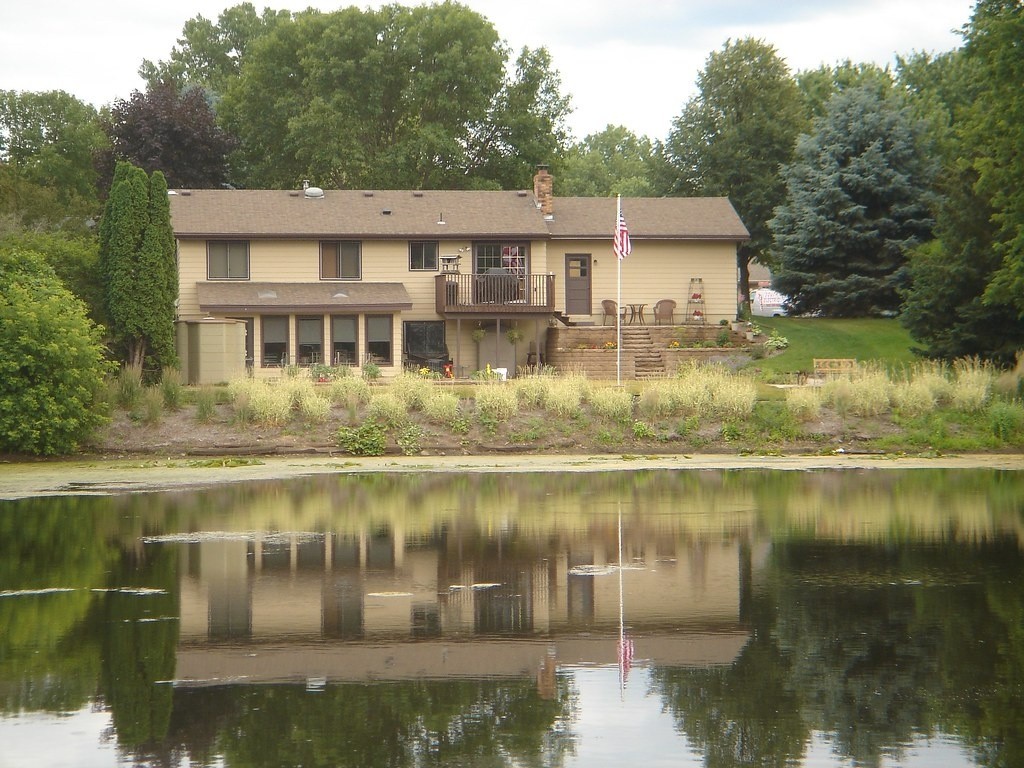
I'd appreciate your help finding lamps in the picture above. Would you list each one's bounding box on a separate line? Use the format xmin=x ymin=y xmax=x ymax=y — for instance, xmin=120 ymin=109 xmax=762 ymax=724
xmin=458 ymin=245 xmax=470 ymax=253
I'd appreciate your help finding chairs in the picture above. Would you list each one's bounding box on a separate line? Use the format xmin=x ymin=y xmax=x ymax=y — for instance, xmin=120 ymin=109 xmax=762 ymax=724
xmin=601 ymin=300 xmax=627 ymax=327
xmin=653 ymin=300 xmax=676 ymax=326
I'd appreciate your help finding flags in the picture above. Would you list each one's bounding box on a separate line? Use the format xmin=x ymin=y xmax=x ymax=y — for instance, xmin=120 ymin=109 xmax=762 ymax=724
xmin=613 ymin=206 xmax=633 ymax=259
xmin=616 ymin=640 xmax=635 ymax=689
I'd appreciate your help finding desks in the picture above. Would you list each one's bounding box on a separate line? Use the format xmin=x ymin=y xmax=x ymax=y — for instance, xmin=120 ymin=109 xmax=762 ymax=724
xmin=527 ymin=352 xmax=545 ymax=367
xmin=626 ymin=303 xmax=648 ymax=326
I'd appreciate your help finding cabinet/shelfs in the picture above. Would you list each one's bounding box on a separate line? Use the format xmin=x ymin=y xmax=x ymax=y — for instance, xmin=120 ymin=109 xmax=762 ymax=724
xmin=686 ymin=277 xmax=707 ymax=325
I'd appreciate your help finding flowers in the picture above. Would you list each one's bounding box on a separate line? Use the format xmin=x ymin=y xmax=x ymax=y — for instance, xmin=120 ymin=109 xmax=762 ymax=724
xmin=693 ymin=310 xmax=703 ymax=316
xmin=692 ymin=294 xmax=701 ymax=299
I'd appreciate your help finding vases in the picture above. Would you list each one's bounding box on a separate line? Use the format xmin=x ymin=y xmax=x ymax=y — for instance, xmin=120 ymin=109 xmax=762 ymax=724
xmin=689 ymin=299 xmax=703 ymax=302
xmin=694 ymin=316 xmax=701 ymax=321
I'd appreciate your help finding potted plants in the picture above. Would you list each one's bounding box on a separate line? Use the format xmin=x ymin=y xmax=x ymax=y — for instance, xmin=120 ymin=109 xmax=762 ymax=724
xmin=746 ymin=328 xmax=754 ymax=339
xmin=506 ymin=328 xmax=526 ymax=344
xmin=732 ymin=321 xmax=739 ymax=331
xmin=471 ymin=329 xmax=487 ymax=342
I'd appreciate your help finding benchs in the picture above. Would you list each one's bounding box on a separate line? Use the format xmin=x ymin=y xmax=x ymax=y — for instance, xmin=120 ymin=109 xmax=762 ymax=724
xmin=812 ymin=358 xmax=858 ymax=378
